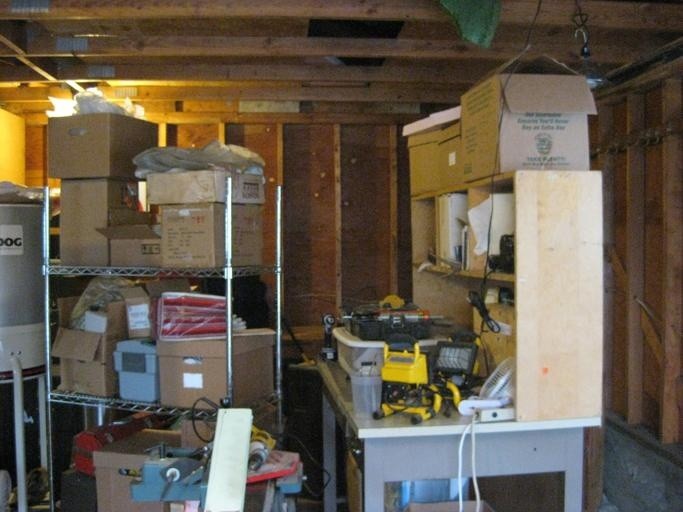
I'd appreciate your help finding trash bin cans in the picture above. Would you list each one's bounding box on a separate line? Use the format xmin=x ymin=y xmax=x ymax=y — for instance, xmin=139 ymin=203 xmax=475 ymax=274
xmin=273 ymin=357 xmax=324 ymax=501
xmin=407 ymin=500 xmax=495 ymax=511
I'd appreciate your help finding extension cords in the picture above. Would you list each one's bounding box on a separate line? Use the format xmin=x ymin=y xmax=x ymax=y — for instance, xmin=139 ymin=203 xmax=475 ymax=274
xmin=475 ymin=409 xmax=516 ymax=423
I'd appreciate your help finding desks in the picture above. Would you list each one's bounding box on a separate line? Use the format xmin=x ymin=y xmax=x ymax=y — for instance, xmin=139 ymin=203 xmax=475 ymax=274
xmin=319 ymin=339 xmax=602 ymax=512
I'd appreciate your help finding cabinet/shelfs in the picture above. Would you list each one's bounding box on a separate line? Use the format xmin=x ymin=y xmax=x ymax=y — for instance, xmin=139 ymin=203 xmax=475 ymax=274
xmin=410 ymin=168 xmax=604 ymax=419
xmin=0 ymin=171 xmax=287 ymax=512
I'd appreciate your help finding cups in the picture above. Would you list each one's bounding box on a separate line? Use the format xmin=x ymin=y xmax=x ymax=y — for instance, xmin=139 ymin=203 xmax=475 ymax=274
xmin=350 ymin=375 xmax=382 ymax=420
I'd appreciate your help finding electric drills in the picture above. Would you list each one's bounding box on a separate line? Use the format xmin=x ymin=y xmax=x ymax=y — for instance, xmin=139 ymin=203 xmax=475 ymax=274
xmin=248 ymin=424 xmax=277 ymax=472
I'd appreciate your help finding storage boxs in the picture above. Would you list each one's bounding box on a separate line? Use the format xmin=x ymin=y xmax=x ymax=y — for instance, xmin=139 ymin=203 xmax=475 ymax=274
xmin=53 ymin=289 xmax=125 ymax=400
xmin=143 ymin=168 xmax=269 ymax=207
xmin=55 ymin=179 xmax=140 ymax=267
xmin=401 ymin=46 xmax=599 ymax=196
xmin=332 ymin=324 xmax=462 ymax=377
xmin=47 ymin=114 xmax=160 ymax=177
xmin=111 ymin=338 xmax=162 ymax=405
xmin=94 ymin=206 xmax=163 ymax=267
xmin=157 ymin=201 xmax=267 ymax=268
xmin=154 ymin=323 xmax=277 ymax=410
xmin=87 ymin=427 xmax=182 ymax=510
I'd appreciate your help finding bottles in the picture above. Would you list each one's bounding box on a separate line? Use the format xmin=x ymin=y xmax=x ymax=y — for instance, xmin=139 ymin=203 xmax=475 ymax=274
xmin=355 ymin=361 xmax=380 ymax=377
